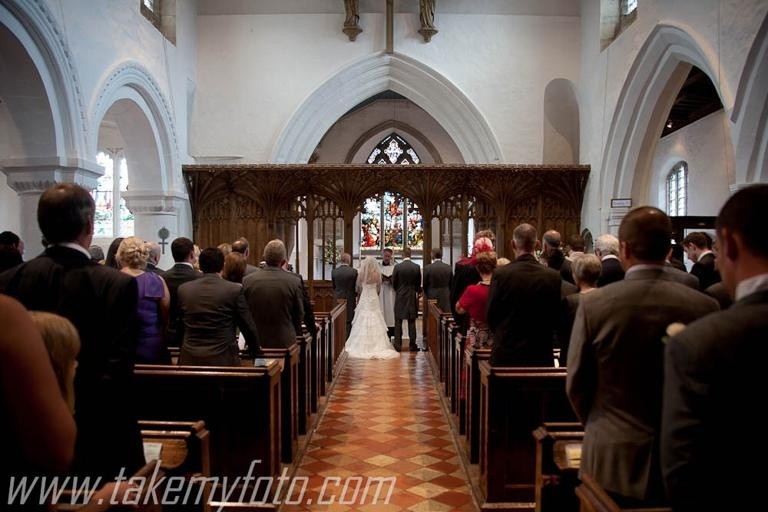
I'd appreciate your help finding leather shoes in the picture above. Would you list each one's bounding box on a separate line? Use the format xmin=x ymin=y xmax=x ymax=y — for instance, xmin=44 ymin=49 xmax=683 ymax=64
xmin=410 ymin=346 xmax=421 ymax=352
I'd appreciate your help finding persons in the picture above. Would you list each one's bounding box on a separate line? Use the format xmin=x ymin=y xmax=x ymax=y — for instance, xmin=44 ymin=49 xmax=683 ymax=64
xmin=1 ymin=231 xmax=23 ymax=255
xmin=275 ymin=247 xmax=318 ymax=337
xmin=115 ymin=239 xmax=170 ymax=359
xmin=145 ymin=242 xmax=165 ymax=278
xmin=331 ymin=253 xmax=357 ymax=341
xmin=224 ymin=251 xmax=247 ymax=281
xmin=161 ymin=237 xmax=201 ymax=347
xmin=496 ymin=257 xmax=511 ymax=268
xmin=566 ymin=252 xmax=604 ymax=313
xmin=422 ymin=247 xmax=452 ymax=318
xmin=593 ymin=234 xmax=622 ymax=283
xmin=177 ymin=247 xmax=261 ymax=367
xmin=540 ymin=248 xmax=564 ymax=271
xmin=242 ymin=240 xmax=308 ymax=349
xmin=343 ymin=258 xmax=398 ymax=364
xmin=0 ymin=170 xmax=147 ymax=476
xmin=487 ymin=224 xmax=562 ymax=371
xmin=375 ymin=249 xmax=397 ymax=344
xmin=0 ymin=246 xmax=22 ymax=275
xmin=28 ymin=310 xmax=159 ymax=512
xmin=230 ymin=240 xmax=257 ymax=273
xmin=567 ymin=230 xmax=585 ymax=252
xmin=566 ymin=204 xmax=719 ymax=510
xmin=541 ymin=224 xmax=563 ymax=271
xmin=0 ymin=294 xmax=77 ymax=461
xmin=391 ymin=247 xmax=421 ymax=351
xmin=676 ymin=230 xmax=724 ymax=290
xmin=666 ymin=183 xmax=763 ymax=512
xmin=455 ymin=251 xmax=495 ymax=400
